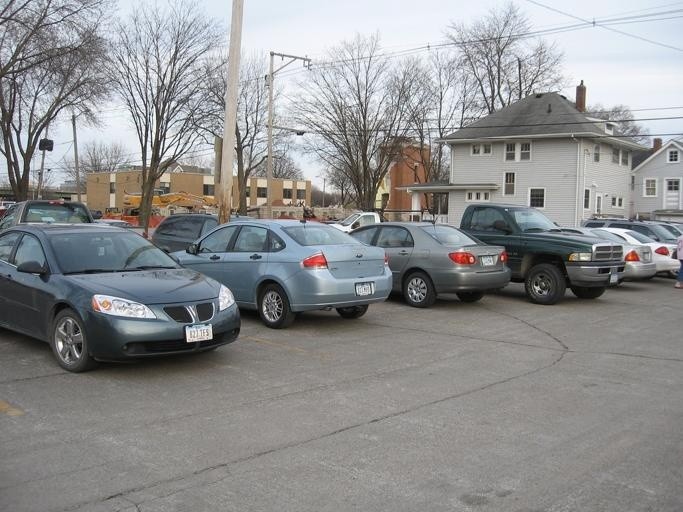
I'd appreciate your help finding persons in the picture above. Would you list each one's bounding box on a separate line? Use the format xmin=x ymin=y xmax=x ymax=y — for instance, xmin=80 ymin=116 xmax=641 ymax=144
xmin=302 ymin=205 xmax=315 ymax=217
xmin=673 ymin=234 xmax=683 ymax=288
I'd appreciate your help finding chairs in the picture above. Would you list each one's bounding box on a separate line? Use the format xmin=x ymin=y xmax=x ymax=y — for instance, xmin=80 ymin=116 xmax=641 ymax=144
xmin=245 ymin=233 xmax=262 ymax=250
xmin=387 ymin=235 xmax=400 ymax=247
xmin=494 ymin=220 xmax=505 ymax=229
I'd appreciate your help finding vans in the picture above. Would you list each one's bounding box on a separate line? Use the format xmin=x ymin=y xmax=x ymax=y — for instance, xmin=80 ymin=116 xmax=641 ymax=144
xmin=0 ymin=198 xmax=95 ymax=231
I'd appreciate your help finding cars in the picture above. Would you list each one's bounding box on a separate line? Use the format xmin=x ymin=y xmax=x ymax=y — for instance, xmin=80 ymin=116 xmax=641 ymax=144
xmin=151 ymin=212 xmax=257 ymax=254
xmin=347 ymin=219 xmax=512 ymax=308
xmin=0 ymin=221 xmax=242 ymax=374
xmin=584 ymin=217 xmax=682 ymax=282
xmin=167 ymin=216 xmax=395 ymax=330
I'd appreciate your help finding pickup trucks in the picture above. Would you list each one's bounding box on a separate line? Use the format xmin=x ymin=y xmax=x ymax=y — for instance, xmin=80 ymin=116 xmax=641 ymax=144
xmin=329 ymin=211 xmax=380 ymax=234
xmin=461 ymin=202 xmax=626 ymax=305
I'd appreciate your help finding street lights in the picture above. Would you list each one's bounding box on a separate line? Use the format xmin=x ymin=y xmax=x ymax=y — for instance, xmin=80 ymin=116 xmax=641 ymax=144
xmin=265 ymin=121 xmax=307 ymax=219
xmin=70 ymin=108 xmax=93 ymax=203
